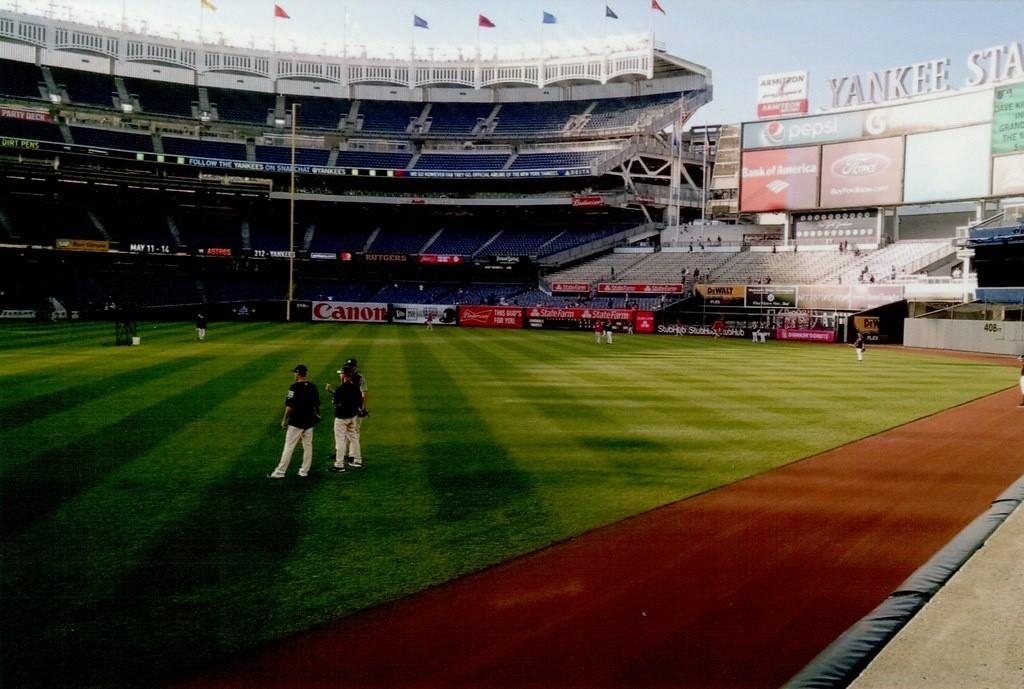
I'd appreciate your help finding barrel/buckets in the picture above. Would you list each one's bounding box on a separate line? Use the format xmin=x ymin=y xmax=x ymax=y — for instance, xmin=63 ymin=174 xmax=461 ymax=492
xmin=132 ymin=337 xmax=141 ymax=345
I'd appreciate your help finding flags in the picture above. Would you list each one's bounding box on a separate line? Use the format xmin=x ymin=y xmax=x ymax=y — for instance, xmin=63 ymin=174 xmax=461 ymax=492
xmin=413 ymin=15 xmax=430 ymax=29
xmin=201 ymin=0 xmax=218 ymax=12
xmin=275 ymin=4 xmax=291 ymax=19
xmin=651 ymin=0 xmax=666 ymax=16
xmin=605 ymin=5 xmax=619 ymax=18
xmin=478 ymin=15 xmax=495 ymax=27
xmin=542 ymin=11 xmax=558 ymax=23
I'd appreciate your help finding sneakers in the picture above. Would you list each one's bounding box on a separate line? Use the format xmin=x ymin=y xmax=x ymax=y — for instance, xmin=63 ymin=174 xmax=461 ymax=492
xmin=295 ymin=474 xmax=308 ymax=480
xmin=327 ymin=454 xmax=336 ymax=460
xmin=326 ymin=467 xmax=346 ymax=472
xmin=267 ymin=473 xmax=271 ymax=479
xmin=347 ymin=461 xmax=362 ymax=467
xmin=347 ymin=457 xmax=354 ymax=462
xmin=1017 ymin=403 xmax=1024 ymax=408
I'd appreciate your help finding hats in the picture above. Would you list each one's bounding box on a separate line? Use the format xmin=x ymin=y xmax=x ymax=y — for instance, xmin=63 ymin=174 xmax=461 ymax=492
xmin=291 ymin=365 xmax=307 ymax=373
xmin=343 ymin=359 xmax=356 ymax=367
xmin=337 ymin=367 xmax=352 ymax=376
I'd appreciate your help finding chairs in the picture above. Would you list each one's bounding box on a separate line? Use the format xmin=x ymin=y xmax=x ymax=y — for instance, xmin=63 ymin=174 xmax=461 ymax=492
xmin=1 ymin=60 xmax=981 ymax=310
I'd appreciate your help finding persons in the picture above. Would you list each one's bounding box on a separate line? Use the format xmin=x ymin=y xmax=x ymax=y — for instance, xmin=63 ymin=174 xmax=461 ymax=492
xmin=717 ymin=235 xmax=723 ymax=243
xmin=267 ymin=365 xmax=323 ymax=480
xmin=680 ymin=266 xmax=771 ymax=285
xmin=772 ymin=243 xmax=776 ymax=253
xmin=196 ymin=313 xmax=207 ymax=340
xmin=297 ymin=182 xmax=361 ymax=195
xmin=853 ymin=333 xmax=865 ymax=360
xmin=688 ymin=237 xmax=694 ymax=253
xmin=424 ymin=287 xmax=548 ymax=332
xmin=838 ymin=263 xmax=929 ymax=284
xmin=326 ymin=367 xmax=361 ymax=472
xmin=794 ymin=244 xmax=798 ymax=253
xmin=1017 ymin=355 xmax=1024 ymax=407
xmin=567 ymin=266 xmax=725 ymax=346
xmin=839 ymin=239 xmax=862 ymax=257
xmin=327 ymin=360 xmax=368 ymax=461
xmin=698 ymin=236 xmax=704 ymax=250
xmin=708 ymin=236 xmax=713 ymax=246
